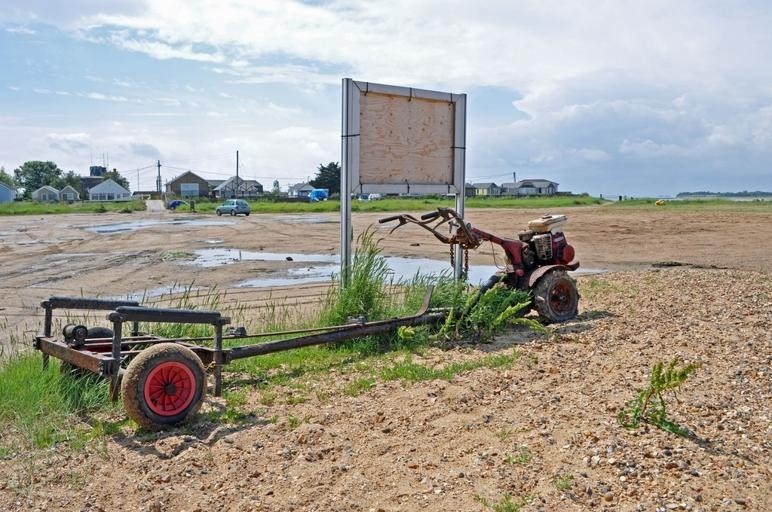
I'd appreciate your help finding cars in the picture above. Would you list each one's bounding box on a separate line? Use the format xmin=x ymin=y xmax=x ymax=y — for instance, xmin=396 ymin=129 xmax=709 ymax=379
xmin=169 ymin=200 xmax=189 ymax=209
xmin=359 ymin=193 xmax=383 ymax=201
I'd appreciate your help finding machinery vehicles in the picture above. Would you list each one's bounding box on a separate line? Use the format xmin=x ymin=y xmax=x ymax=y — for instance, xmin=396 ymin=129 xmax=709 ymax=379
xmin=32 ymin=205 xmax=582 ymax=431
xmin=216 ymin=198 xmax=250 ymax=216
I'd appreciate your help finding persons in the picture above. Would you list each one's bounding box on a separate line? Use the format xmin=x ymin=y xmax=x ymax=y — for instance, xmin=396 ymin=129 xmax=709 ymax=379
xmin=184 ymin=192 xmax=197 ymax=213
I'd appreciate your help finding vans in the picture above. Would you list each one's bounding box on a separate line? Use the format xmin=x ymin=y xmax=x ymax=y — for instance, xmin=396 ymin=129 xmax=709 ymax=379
xmin=310 ymin=189 xmax=328 ymax=203
